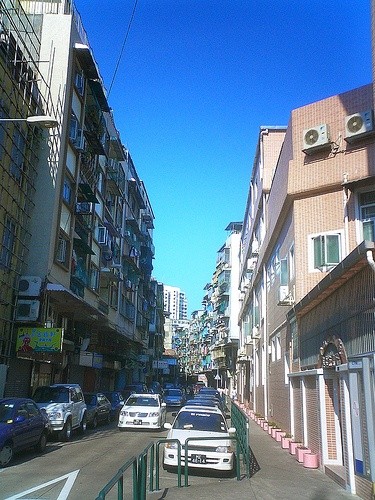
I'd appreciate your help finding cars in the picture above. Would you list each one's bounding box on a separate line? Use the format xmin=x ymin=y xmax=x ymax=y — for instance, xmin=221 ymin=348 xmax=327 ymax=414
xmin=0 ymin=397 xmax=53 ymax=469
xmin=118 ymin=394 xmax=167 ymax=432
xmin=163 ymin=406 xmax=237 ymax=477
xmin=84 ymin=392 xmax=112 ymax=430
xmin=102 ymin=382 xmax=230 ymax=420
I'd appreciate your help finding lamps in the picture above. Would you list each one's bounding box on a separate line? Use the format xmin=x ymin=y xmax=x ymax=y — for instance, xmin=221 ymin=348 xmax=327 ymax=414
xmin=126 ymin=281 xmax=138 ymax=293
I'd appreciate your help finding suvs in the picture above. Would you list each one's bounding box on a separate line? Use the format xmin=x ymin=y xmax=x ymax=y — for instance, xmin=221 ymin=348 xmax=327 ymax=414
xmin=31 ymin=383 xmax=87 ymax=442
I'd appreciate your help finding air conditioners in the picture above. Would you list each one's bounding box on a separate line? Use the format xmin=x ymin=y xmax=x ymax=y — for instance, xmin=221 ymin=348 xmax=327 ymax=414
xmin=236 ymin=327 xmax=260 ymax=359
xmin=17 ymin=277 xmax=42 ymax=298
xmin=300 ymin=124 xmax=332 ymax=155
xmin=12 ymin=300 xmax=41 ymax=323
xmin=277 ymin=286 xmax=290 ymax=301
xmin=238 ymin=241 xmax=259 ymax=303
xmin=343 ymin=106 xmax=374 ymax=141
